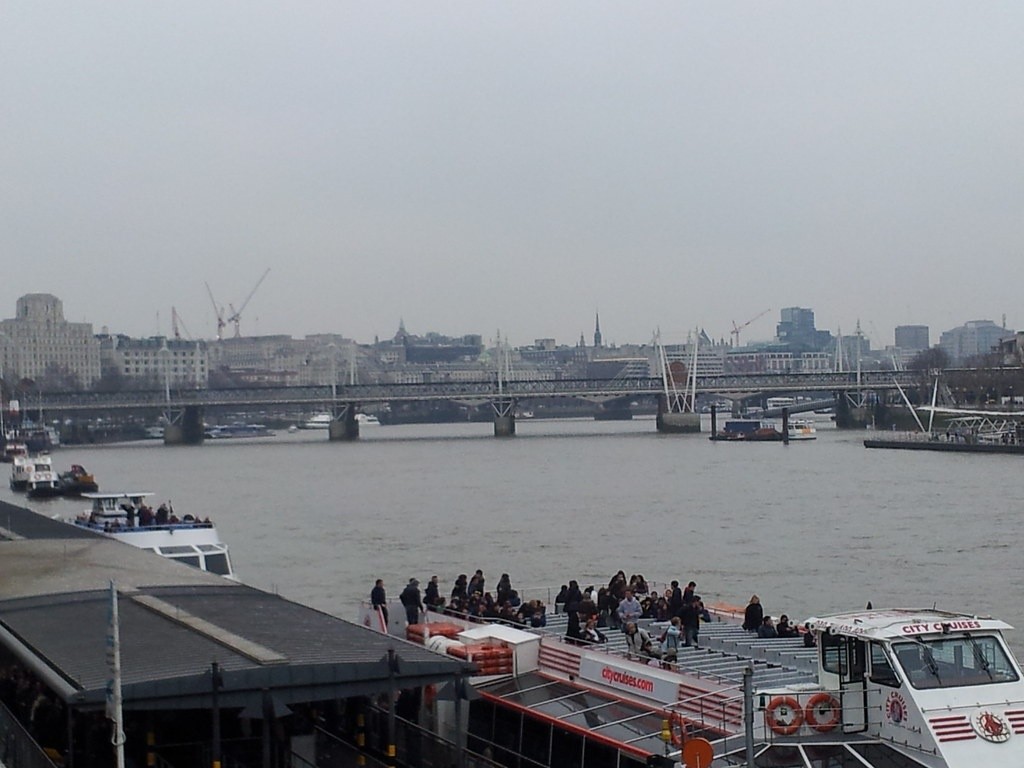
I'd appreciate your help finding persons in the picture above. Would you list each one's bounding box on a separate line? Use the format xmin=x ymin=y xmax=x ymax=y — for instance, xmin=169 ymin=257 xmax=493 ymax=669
xmin=553 ymin=569 xmax=710 ymax=664
xmin=88 ymin=503 xmax=213 ymax=533
xmin=741 ymin=594 xmax=763 ymax=631
xmin=399 ymin=577 xmax=425 ymax=625
xmin=757 ymin=614 xmax=799 ymax=638
xmin=370 ymin=578 xmax=389 ymax=633
xmin=423 ymin=569 xmax=547 ymax=631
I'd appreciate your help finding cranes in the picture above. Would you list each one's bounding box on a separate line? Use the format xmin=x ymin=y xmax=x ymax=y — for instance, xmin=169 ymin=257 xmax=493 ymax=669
xmin=729 ymin=307 xmax=772 ymax=346
xmin=202 ymin=282 xmax=226 ymax=339
xmin=228 ymin=266 xmax=271 ymax=336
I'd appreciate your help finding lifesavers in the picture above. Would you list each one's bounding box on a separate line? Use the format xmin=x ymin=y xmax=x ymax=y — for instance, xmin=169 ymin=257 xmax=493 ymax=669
xmin=766 ymin=695 xmax=803 ymax=733
xmin=422 ymin=683 xmax=436 ymax=707
xmin=770 ymin=741 xmax=798 ymax=763
xmin=665 ymin=713 xmax=688 ymax=743
xmin=801 ymin=691 xmax=844 ymax=732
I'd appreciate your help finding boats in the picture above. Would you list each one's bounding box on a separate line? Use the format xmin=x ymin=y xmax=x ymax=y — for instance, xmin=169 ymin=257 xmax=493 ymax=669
xmin=0 ymin=390 xmax=61 ymax=462
xmin=9 ymin=454 xmax=59 ymax=492
xmin=52 ymin=494 xmax=236 ymax=581
xmin=357 ymin=582 xmax=1024 ymax=768
xmin=57 ymin=465 xmax=99 ymax=494
xmin=724 ymin=418 xmax=818 ymax=440
xmin=863 ymin=425 xmax=1024 ymax=454
xmin=708 ymin=428 xmax=782 ymax=441
